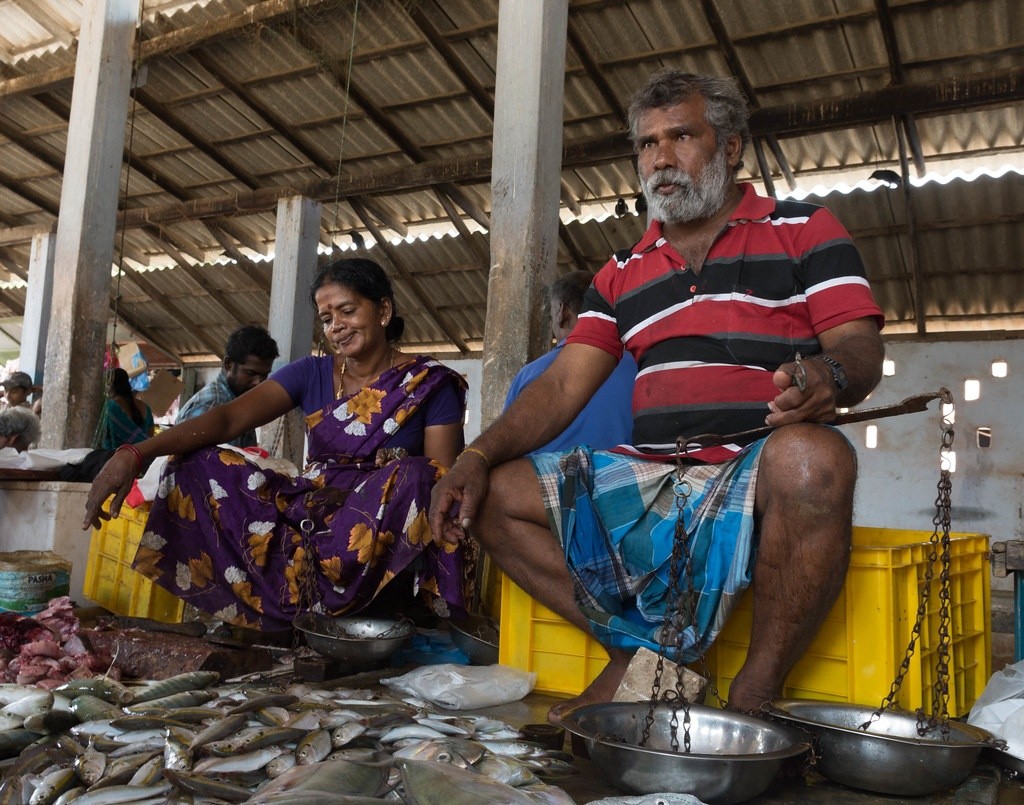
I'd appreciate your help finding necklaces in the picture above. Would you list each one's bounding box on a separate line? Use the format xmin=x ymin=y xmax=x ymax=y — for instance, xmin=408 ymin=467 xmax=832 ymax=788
xmin=337 ymin=349 xmax=395 ymax=399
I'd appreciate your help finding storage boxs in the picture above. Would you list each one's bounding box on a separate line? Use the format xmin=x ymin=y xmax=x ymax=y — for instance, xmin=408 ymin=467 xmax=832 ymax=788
xmin=0 ymin=480 xmax=101 ymax=610
xmin=496 ymin=566 xmax=612 ymax=701
xmin=82 ymin=495 xmax=189 ymax=626
xmin=698 ymin=521 xmax=994 ymax=720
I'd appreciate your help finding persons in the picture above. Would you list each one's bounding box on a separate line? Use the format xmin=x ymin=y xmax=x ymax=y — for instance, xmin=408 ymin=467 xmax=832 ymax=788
xmin=0 ymin=371 xmax=33 ymax=411
xmin=83 ymin=257 xmax=481 ymax=668
xmin=101 ymin=366 xmax=154 ymax=450
xmin=502 ymin=270 xmax=638 ymax=455
xmin=174 ymin=326 xmax=280 ymax=449
xmin=431 ymin=71 xmax=887 ymax=724
xmin=0 ymin=405 xmax=43 ymax=454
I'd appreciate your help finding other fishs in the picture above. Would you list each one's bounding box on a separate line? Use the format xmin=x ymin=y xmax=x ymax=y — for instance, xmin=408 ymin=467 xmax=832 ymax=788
xmin=0 ymin=670 xmax=711 ymax=805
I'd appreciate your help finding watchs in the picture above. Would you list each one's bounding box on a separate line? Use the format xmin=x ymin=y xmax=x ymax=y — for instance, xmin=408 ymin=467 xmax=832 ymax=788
xmin=817 ymin=355 xmax=849 ymax=394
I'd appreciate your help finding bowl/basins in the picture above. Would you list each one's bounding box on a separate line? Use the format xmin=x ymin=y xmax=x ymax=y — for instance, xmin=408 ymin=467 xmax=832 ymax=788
xmin=760 ymin=698 xmax=1004 ymax=797
xmin=560 ymin=701 xmax=811 ymax=804
xmin=292 ymin=616 xmax=417 ymax=661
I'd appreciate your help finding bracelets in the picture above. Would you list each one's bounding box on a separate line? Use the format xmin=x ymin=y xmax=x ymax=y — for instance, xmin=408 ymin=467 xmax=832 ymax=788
xmin=456 ymin=448 xmax=490 ymax=467
xmin=116 ymin=443 xmax=142 ymax=475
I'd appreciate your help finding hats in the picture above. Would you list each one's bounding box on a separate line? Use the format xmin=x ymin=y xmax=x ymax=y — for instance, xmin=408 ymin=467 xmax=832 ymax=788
xmin=1 ymin=370 xmax=34 ymax=392
